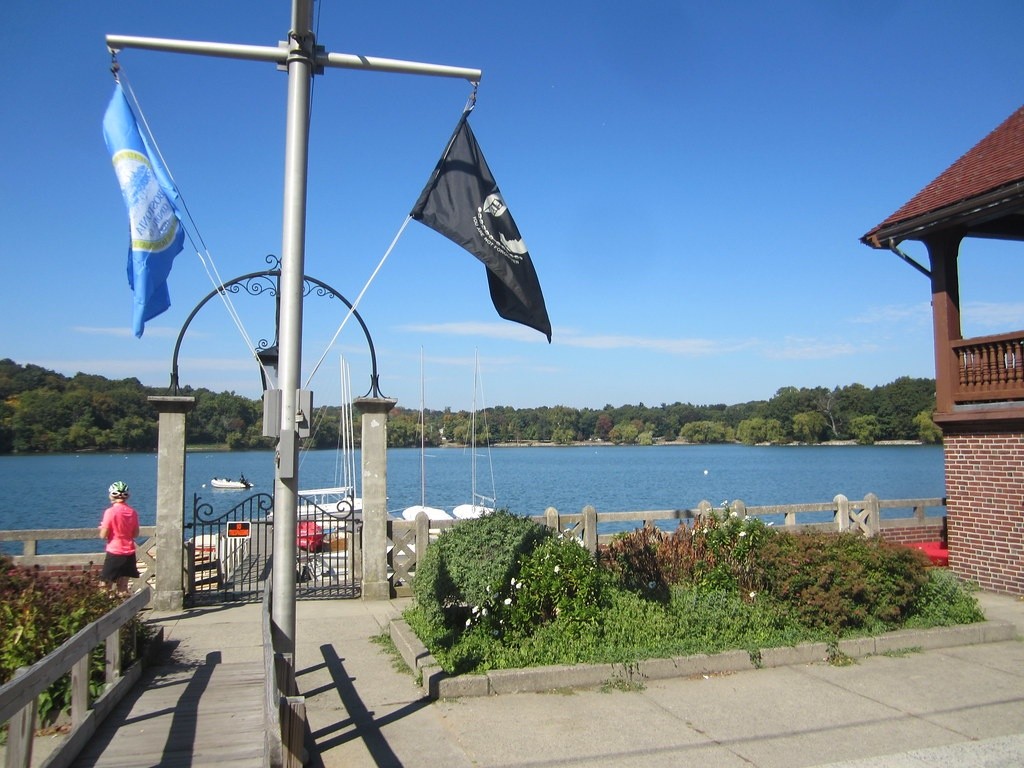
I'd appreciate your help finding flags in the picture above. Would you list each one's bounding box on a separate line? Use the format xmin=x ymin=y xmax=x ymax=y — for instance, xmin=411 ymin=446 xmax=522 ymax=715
xmin=102 ymin=85 xmax=184 ymax=340
xmin=412 ymin=120 xmax=552 ymax=343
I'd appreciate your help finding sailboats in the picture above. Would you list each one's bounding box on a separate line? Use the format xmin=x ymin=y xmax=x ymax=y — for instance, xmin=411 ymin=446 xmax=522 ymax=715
xmin=269 ymin=353 xmax=390 ymax=518
xmin=452 ymin=347 xmax=496 ymax=520
xmin=402 ymin=343 xmax=454 ymax=536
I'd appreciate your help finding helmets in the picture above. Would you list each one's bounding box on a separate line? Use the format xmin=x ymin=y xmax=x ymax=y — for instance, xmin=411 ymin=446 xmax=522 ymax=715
xmin=109 ymin=480 xmax=130 ymax=500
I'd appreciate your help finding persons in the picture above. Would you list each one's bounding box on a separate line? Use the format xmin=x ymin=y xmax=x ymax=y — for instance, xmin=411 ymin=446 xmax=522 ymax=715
xmin=99 ymin=481 xmax=139 ymax=592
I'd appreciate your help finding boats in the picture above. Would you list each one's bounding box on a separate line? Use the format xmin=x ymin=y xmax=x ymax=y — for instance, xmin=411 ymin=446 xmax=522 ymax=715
xmin=211 ymin=471 xmax=255 ymax=488
